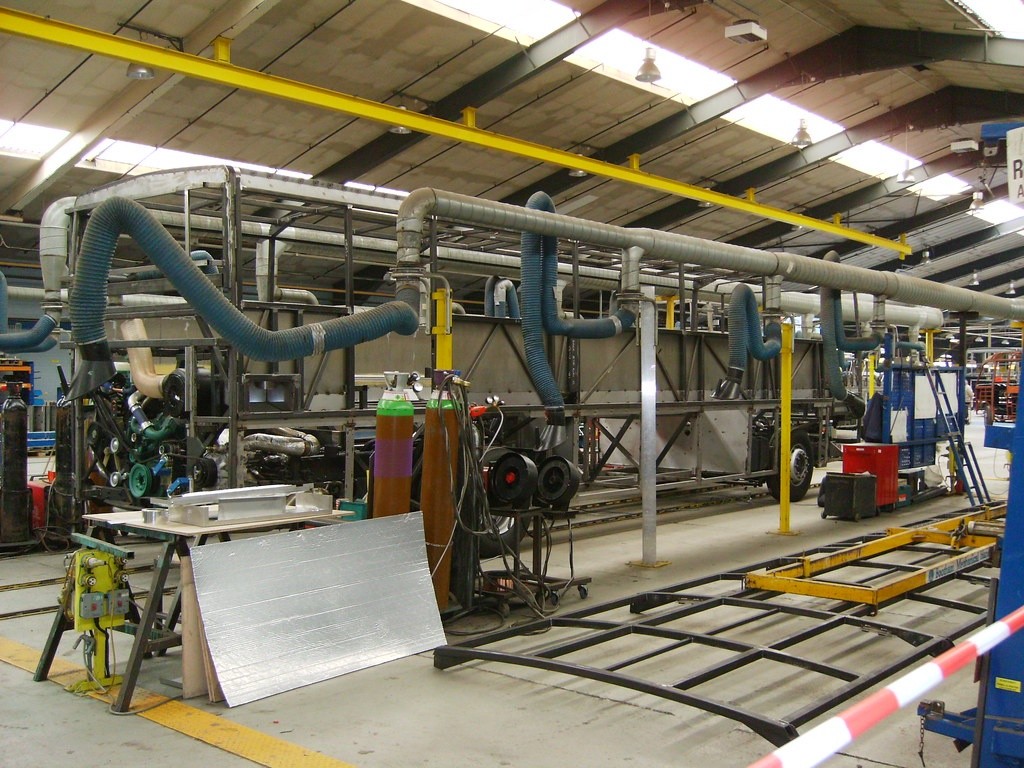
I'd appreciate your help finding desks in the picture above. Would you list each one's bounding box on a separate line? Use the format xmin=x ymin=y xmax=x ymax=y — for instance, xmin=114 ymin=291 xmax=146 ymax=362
xmin=32 ymin=505 xmax=355 ymax=713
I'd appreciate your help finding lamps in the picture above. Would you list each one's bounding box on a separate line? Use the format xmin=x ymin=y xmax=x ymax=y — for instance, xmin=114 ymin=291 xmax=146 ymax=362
xmin=388 ymin=90 xmax=436 ymax=135
xmin=635 ymin=0 xmax=662 ymax=83
xmin=117 ymin=22 xmax=183 ymax=79
xmin=920 ymin=242 xmax=935 ymax=265
xmin=791 ymin=204 xmax=811 ymax=230
xmin=969 ymin=269 xmax=980 ymax=285
xmin=569 ymin=141 xmax=602 ymax=177
xmin=1005 ymin=279 xmax=1017 ymax=295
xmin=897 ymin=124 xmax=914 ymax=183
xmin=698 ymin=176 xmax=723 ymax=208
xmin=789 ymin=71 xmax=813 ymax=145
xmin=970 ymin=162 xmax=984 ymax=210
